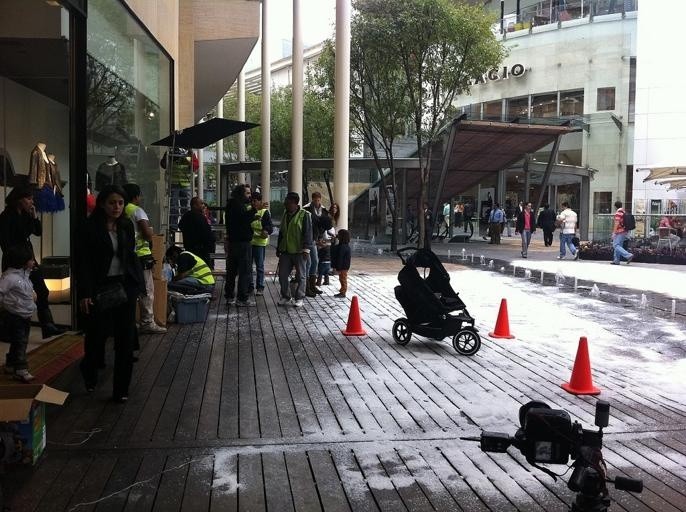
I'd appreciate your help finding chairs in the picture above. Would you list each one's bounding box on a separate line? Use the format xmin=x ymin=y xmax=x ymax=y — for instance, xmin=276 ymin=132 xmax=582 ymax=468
xmin=656 ymin=226 xmax=672 ymax=250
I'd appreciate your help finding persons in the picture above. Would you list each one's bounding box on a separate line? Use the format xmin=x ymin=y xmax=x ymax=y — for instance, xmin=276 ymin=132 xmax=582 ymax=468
xmin=120 ymin=184 xmax=169 ymax=335
xmin=75 ymin=183 xmax=148 ymax=402
xmin=24 ymin=140 xmax=130 ymax=214
xmin=277 ymin=192 xmax=351 ymax=306
xmin=166 ymin=184 xmax=274 ymax=308
xmin=0 ymin=243 xmax=38 ymax=383
xmin=404 ymin=198 xmax=578 ymax=261
xmin=0 ymin=186 xmax=69 ymax=344
xmin=158 ymin=147 xmax=200 ymax=233
xmin=659 ymin=210 xmax=681 ymax=251
xmin=609 ymin=201 xmax=634 ymax=263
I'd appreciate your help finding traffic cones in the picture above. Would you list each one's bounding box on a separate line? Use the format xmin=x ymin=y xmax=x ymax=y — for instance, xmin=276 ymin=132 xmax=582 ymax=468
xmin=341 ymin=295 xmax=367 ymax=336
xmin=562 ymin=336 xmax=601 ymax=395
xmin=488 ymin=298 xmax=516 ymax=339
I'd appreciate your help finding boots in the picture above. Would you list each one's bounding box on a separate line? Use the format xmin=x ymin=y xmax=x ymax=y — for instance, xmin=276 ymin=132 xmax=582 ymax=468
xmin=323 ymin=277 xmax=329 ymax=285
xmin=310 ymin=274 xmax=322 ymax=294
xmin=316 ymin=277 xmax=320 ymax=285
xmin=306 ymin=277 xmax=315 ymax=296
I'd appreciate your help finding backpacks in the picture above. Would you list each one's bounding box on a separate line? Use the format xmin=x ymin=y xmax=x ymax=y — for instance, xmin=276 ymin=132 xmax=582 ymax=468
xmin=618 ymin=211 xmax=635 ymax=230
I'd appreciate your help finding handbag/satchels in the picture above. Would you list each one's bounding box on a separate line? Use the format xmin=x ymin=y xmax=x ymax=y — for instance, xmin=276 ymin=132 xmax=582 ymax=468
xmin=92 ymin=283 xmax=128 ymax=310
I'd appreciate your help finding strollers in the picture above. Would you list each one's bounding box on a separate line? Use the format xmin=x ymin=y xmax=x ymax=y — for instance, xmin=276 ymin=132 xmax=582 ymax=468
xmin=392 ymin=247 xmax=480 ymax=354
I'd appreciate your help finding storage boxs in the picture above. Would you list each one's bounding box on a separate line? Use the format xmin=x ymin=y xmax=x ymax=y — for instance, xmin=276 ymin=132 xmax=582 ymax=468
xmin=0 ymin=384 xmax=68 ymax=466
xmin=171 ymin=297 xmax=210 ymax=324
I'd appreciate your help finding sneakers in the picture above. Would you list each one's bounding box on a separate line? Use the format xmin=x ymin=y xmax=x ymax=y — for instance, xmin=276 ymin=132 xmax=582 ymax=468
xmin=556 ymin=256 xmax=564 ymax=260
xmin=334 ymin=294 xmax=345 ymax=297
xmin=227 ymin=291 xmax=305 ymax=306
xmin=11 ymin=369 xmax=33 ymax=382
xmin=522 ymin=252 xmax=527 ymax=258
xmin=572 ymin=250 xmax=579 ymax=259
xmin=140 ymin=321 xmax=167 ymax=334
xmin=610 ymin=262 xmax=620 ymax=265
xmin=627 ymin=255 xmax=633 ymax=264
xmin=43 ymin=328 xmax=67 ymax=338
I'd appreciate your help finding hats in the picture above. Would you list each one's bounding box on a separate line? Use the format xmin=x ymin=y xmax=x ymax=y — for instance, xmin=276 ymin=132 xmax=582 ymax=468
xmin=6 ymin=183 xmax=32 ymax=203
